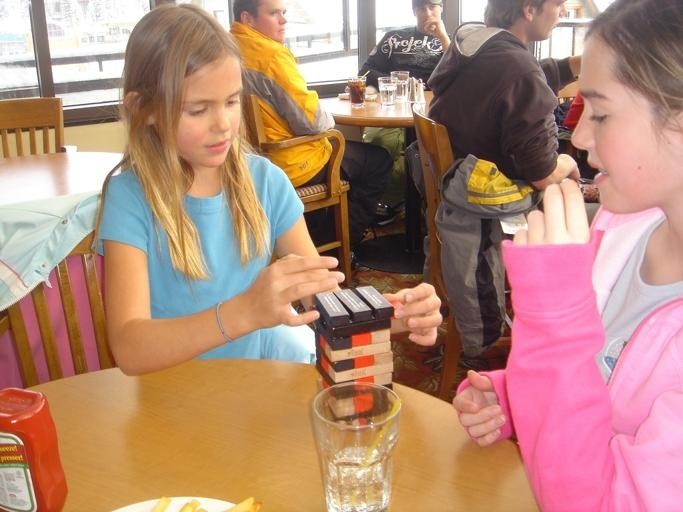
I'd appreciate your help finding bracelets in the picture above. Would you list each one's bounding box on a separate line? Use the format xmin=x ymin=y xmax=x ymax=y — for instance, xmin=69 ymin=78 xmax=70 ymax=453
xmin=214 ymin=300 xmax=234 ymax=343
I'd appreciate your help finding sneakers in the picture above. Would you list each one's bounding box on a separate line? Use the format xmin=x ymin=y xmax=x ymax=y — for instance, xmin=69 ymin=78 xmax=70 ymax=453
xmin=371 ymin=200 xmax=401 ymax=227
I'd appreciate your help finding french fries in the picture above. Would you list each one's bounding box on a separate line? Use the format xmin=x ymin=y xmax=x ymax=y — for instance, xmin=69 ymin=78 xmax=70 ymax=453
xmin=149 ymin=495 xmax=262 ymax=512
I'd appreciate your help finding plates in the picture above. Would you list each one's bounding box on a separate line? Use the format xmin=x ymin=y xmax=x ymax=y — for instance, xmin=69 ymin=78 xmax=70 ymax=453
xmin=337 ymin=93 xmax=377 ymax=102
xmin=105 ymin=497 xmax=235 ymax=512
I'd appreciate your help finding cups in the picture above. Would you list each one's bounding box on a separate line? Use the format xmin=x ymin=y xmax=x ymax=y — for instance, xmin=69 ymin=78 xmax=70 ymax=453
xmin=348 ymin=75 xmax=366 ymax=108
xmin=309 ymin=381 xmax=401 ymax=512
xmin=376 ymin=69 xmax=409 ymax=106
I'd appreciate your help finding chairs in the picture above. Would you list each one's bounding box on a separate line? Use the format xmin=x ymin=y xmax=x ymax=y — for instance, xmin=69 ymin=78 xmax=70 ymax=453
xmin=0 ymin=193 xmax=117 ymax=387
xmin=409 ymin=99 xmax=511 ymax=403
xmin=0 ymin=96 xmax=65 ymax=159
xmin=242 ymin=93 xmax=354 ymax=290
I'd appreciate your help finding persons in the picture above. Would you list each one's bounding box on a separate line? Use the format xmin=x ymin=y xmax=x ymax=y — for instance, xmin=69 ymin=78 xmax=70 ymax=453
xmin=357 ymin=0 xmax=455 ymax=91
xmin=424 ymin=0 xmax=583 ymax=191
xmin=453 ymin=0 xmax=681 ymax=512
xmin=226 ymin=0 xmax=392 ymax=270
xmin=89 ymin=3 xmax=446 ymax=377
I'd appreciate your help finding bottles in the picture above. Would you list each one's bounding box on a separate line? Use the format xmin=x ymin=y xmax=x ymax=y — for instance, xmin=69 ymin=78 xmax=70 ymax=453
xmin=408 ymin=76 xmax=425 ymax=105
